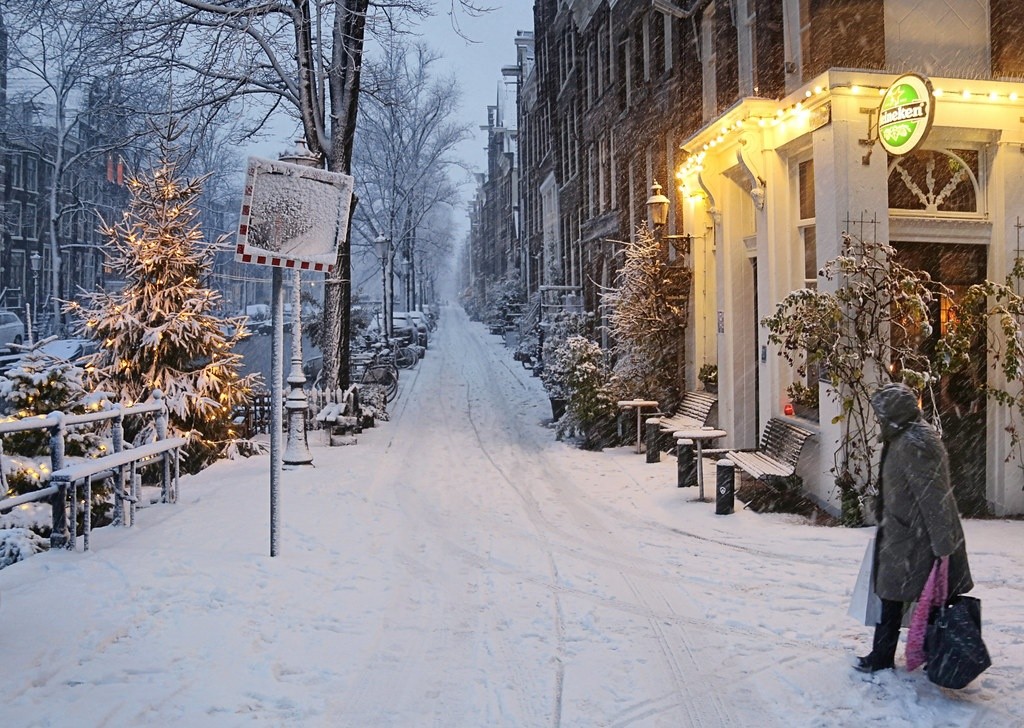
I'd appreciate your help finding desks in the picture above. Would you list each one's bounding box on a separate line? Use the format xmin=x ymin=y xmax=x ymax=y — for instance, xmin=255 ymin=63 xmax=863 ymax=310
xmin=672 ymin=429 xmax=727 ymax=504
xmin=619 ymin=399 xmax=658 ymax=454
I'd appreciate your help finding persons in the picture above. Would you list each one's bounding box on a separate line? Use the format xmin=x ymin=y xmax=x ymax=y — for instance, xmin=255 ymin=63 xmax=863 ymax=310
xmin=853 ymin=382 xmax=975 ymax=677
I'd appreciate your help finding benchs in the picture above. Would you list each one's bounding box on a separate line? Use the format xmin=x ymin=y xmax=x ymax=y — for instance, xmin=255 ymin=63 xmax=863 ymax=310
xmin=725 ymin=418 xmax=815 ymax=511
xmin=315 ymin=402 xmax=348 ymax=447
xmin=659 ymin=389 xmax=717 ymax=455
xmin=52 ymin=437 xmax=186 ymax=554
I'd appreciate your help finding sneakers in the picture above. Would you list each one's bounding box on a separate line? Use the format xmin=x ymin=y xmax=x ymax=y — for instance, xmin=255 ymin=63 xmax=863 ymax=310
xmin=851 ymin=651 xmax=896 ymax=670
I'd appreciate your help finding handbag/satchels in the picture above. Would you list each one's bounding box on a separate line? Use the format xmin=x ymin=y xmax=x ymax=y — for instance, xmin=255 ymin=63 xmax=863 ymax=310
xmin=905 ymin=558 xmax=992 ymax=689
xmin=847 ymin=530 xmax=883 ymax=626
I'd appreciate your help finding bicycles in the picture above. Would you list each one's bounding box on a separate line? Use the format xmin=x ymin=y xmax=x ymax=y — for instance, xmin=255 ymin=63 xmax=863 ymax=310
xmin=303 ymin=332 xmax=419 ymax=404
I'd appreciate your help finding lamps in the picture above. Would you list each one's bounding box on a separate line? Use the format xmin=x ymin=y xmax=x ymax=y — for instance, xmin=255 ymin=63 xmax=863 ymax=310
xmin=646 ymin=179 xmax=690 ymax=255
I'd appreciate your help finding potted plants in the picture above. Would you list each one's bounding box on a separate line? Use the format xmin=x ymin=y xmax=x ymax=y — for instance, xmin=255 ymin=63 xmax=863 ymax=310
xmin=697 ymin=364 xmax=718 ymax=393
xmin=787 ymin=381 xmax=819 ymax=422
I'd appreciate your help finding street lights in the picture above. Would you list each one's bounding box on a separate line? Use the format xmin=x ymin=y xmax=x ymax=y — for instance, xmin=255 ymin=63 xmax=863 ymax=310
xmin=417 ymin=271 xmax=425 ymax=312
xmin=374 ymin=231 xmax=389 ymax=349
xmin=401 ymin=257 xmax=411 ymax=312
xmin=273 ymin=129 xmax=323 ymax=470
xmin=310 ymin=282 xmax=315 ymax=299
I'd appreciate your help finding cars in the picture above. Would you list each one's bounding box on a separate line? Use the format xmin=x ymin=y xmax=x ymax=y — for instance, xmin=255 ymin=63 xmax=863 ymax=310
xmin=0 ymin=311 xmax=25 ymax=353
xmin=366 ymin=312 xmax=419 ymax=346
xmin=219 ymin=299 xmax=400 ymax=343
xmin=408 ymin=312 xmax=428 ymax=350
xmin=414 ymin=298 xmax=445 ymax=333
